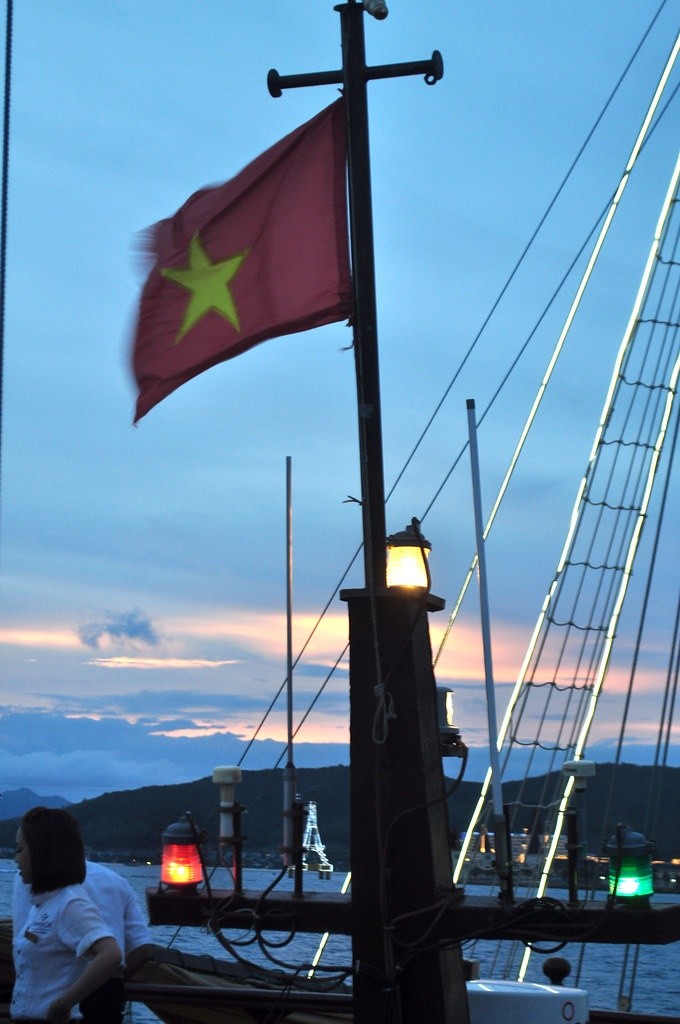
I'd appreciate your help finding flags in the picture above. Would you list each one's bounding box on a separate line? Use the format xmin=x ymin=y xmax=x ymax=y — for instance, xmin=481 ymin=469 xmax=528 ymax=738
xmin=132 ymin=96 xmax=353 ymax=426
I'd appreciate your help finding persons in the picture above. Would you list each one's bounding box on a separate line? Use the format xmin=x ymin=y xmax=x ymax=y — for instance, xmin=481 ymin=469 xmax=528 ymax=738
xmin=14 ymin=859 xmax=153 ymax=1024
xmin=9 ymin=806 xmax=123 ymax=1024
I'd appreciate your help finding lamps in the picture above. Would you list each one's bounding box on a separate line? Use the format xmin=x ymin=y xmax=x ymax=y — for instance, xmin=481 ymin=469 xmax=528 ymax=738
xmin=389 ymin=520 xmax=434 ymax=592
xmin=606 ymin=824 xmax=656 ymax=898
xmin=162 ymin=820 xmax=206 ymax=886
